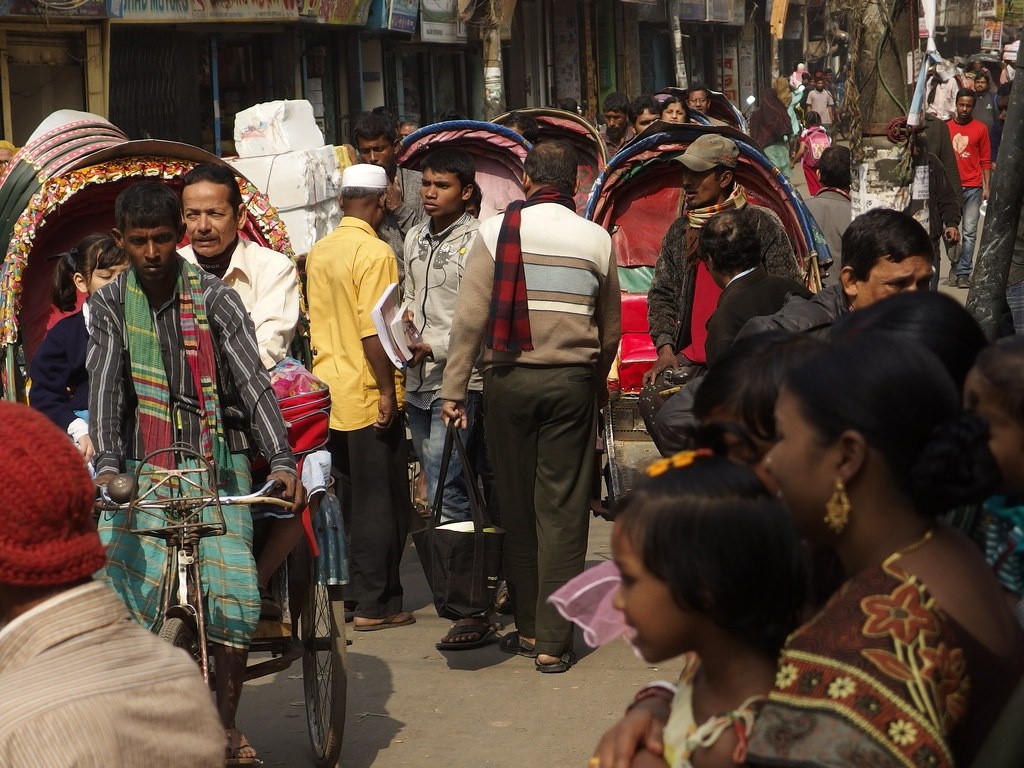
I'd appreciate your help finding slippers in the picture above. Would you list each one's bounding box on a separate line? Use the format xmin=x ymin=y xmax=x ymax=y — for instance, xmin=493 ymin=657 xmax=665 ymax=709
xmin=354 ymin=608 xmax=415 ymax=631
xmin=434 ymin=621 xmax=498 ymax=649
xmin=221 ymin=727 xmax=256 ymax=764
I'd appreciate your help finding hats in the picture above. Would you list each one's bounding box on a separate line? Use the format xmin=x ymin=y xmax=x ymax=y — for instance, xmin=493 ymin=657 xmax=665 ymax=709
xmin=667 ymin=134 xmax=739 ymax=172
xmin=341 ymin=163 xmax=386 ymax=188
xmin=0 ymin=399 xmax=104 ymax=584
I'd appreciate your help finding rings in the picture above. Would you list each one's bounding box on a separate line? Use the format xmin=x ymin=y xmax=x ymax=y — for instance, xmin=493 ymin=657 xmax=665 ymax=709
xmin=590 ymin=758 xmax=600 ymax=765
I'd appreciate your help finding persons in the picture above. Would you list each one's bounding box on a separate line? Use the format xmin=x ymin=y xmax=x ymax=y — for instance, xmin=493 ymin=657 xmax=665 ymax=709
xmin=402 ymin=147 xmax=494 ymax=652
xmin=986 ymin=30 xmax=991 ymax=38
xmin=638 ymin=134 xmax=804 ymax=451
xmin=306 ymin=164 xmax=417 ymax=632
xmin=82 ymin=180 xmax=308 ymax=764
xmin=441 ymin=140 xmax=621 ymax=672
xmin=0 ymin=63 xmax=1024 ymax=768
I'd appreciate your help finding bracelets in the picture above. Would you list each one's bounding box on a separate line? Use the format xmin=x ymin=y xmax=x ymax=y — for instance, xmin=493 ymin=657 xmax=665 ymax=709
xmin=635 ymin=680 xmax=676 ymax=695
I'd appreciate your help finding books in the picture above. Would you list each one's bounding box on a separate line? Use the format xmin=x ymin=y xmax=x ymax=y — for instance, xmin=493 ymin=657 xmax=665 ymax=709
xmin=371 ymin=283 xmax=408 ymax=371
xmin=390 ymin=305 xmax=424 ymax=362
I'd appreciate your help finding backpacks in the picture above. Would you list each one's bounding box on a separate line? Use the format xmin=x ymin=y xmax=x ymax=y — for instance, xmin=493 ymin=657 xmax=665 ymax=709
xmin=801 ymin=128 xmax=831 ymax=164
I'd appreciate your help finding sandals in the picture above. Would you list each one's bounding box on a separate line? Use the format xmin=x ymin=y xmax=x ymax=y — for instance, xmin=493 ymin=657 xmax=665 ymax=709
xmin=490 ymin=581 xmax=513 ymax=614
xmin=535 ymin=653 xmax=571 ymax=673
xmin=502 ymin=631 xmax=538 ymax=658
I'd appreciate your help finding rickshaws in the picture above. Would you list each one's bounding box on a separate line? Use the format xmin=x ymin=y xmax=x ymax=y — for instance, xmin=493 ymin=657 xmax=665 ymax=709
xmin=387 ymin=86 xmax=833 ymax=522
xmin=1 ymin=110 xmax=355 ymax=768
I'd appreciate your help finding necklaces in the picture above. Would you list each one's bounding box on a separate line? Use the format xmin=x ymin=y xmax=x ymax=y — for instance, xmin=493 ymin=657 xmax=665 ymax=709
xmin=886 ymin=528 xmax=933 ymax=564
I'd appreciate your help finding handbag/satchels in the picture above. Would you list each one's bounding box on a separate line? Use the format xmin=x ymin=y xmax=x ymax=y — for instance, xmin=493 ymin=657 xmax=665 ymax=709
xmin=248 ymin=359 xmax=333 ymax=469
xmin=415 ymin=417 xmax=507 ymax=614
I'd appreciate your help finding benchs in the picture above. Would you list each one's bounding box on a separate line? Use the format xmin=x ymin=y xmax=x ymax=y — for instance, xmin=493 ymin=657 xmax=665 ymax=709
xmin=620 ymin=293 xmax=661 ymax=392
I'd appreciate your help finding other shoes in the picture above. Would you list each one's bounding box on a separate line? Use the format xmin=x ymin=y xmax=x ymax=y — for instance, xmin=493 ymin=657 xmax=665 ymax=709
xmin=258 ymin=588 xmax=282 ymax=619
xmin=949 ymin=264 xmax=960 ymax=285
xmin=957 ymin=276 xmax=972 ymax=288
